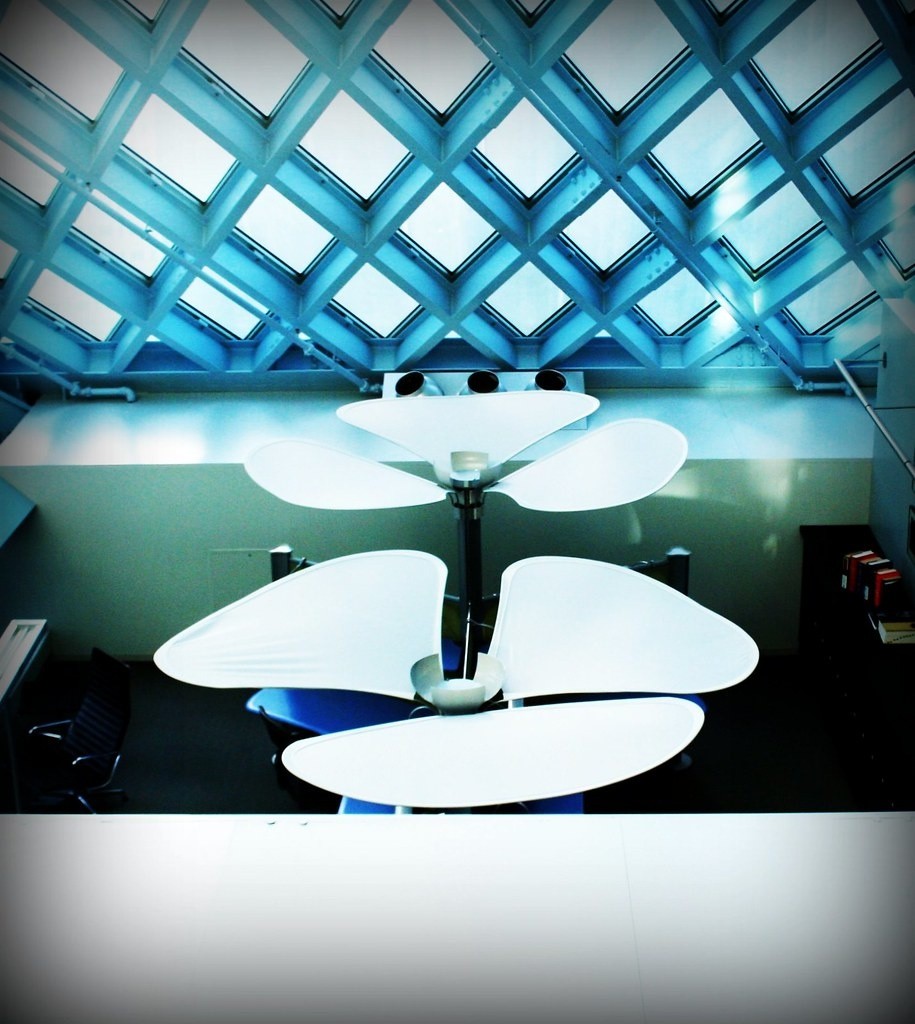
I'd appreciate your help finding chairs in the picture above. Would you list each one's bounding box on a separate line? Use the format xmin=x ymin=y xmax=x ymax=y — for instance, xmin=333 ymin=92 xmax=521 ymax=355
xmin=21 ymin=646 xmax=133 ymax=814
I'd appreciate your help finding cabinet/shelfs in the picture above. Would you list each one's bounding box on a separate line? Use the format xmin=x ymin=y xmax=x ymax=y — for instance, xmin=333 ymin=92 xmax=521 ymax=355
xmin=795 ymin=527 xmax=915 ymax=812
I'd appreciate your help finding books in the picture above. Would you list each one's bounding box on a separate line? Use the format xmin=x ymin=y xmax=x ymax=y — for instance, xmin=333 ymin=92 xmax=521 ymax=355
xmin=840 ymin=550 xmax=915 ymax=645
